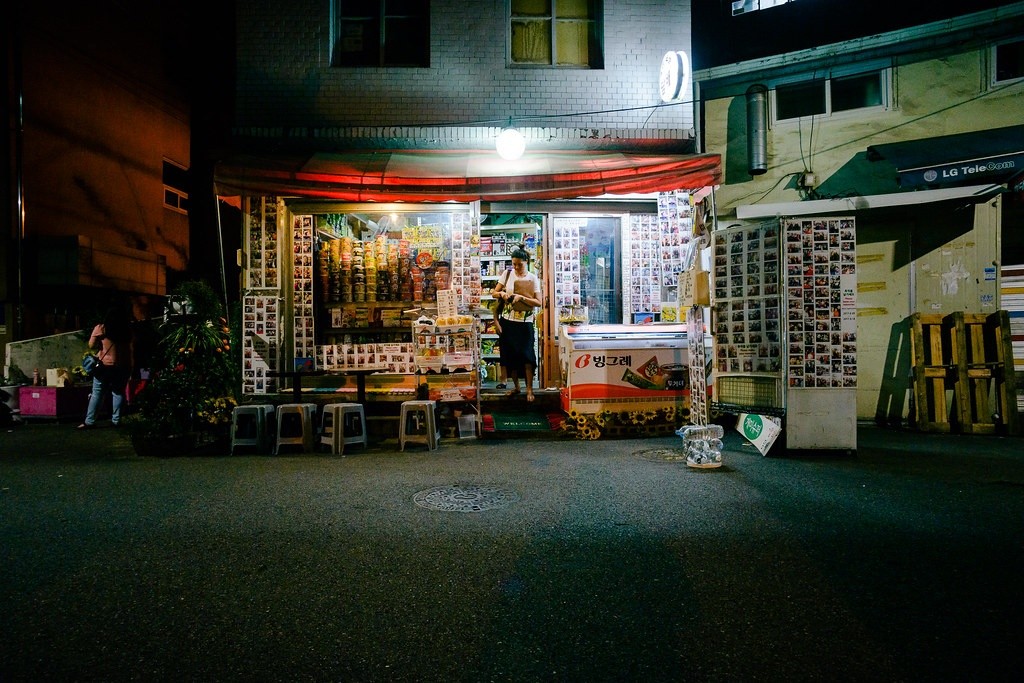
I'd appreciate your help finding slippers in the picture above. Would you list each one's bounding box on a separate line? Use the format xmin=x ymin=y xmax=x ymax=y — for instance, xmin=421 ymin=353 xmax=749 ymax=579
xmin=496 ymin=384 xmax=506 ymax=389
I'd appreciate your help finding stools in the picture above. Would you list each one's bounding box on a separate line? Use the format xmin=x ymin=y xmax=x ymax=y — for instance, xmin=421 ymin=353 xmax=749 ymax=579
xmin=398 ymin=400 xmax=441 ymax=452
xmin=272 ymin=404 xmax=319 ymax=457
xmin=320 ymin=402 xmax=367 ymax=455
xmin=229 ymin=404 xmax=275 ymax=456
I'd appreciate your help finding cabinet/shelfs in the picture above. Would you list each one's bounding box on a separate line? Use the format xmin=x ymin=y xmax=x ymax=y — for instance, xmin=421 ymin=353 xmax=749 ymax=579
xmin=320 ymin=302 xmax=446 ymax=344
xmin=411 ymin=317 xmax=482 ymax=437
xmin=317 ymin=213 xmax=371 ymax=242
xmin=478 ymin=223 xmax=539 ymax=387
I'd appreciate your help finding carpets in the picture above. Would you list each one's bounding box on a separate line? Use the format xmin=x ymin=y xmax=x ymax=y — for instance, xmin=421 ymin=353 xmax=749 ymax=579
xmin=491 ymin=412 xmax=552 ymax=432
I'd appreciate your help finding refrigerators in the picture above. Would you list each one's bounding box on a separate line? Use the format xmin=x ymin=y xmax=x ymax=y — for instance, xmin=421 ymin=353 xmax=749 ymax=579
xmin=558 ymin=324 xmax=713 ymax=414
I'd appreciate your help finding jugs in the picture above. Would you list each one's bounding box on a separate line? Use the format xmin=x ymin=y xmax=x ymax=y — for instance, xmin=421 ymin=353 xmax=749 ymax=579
xmin=457 ymin=414 xmax=476 ymax=438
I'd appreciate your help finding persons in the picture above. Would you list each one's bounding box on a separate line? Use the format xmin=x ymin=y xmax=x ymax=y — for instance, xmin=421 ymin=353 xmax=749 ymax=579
xmin=491 ymin=244 xmax=544 ymax=401
xmin=78 ymin=303 xmax=134 ymax=429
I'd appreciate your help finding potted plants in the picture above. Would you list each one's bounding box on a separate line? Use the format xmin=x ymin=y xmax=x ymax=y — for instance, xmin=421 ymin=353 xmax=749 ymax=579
xmin=116 ymin=411 xmax=172 ymax=456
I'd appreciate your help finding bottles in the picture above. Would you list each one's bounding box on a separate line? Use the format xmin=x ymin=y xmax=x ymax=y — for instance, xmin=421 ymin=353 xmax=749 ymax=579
xmin=32 ymin=369 xmax=40 ymax=385
xmin=674 ymin=424 xmax=724 ymax=464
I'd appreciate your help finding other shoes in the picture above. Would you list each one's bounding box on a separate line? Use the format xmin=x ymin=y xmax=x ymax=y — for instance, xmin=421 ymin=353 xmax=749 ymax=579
xmin=76 ymin=422 xmax=93 ymax=430
xmin=506 ymin=389 xmax=520 ymax=395
xmin=112 ymin=425 xmax=120 ymax=428
xmin=526 ymin=393 xmax=536 ymax=403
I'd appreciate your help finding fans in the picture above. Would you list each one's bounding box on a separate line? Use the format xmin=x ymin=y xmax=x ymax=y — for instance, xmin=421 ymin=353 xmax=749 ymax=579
xmin=366 ymin=217 xmax=391 ymax=240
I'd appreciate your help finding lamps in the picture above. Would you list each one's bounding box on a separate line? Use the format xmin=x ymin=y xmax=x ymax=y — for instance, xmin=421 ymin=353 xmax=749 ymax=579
xmin=496 ymin=116 xmax=526 ymax=160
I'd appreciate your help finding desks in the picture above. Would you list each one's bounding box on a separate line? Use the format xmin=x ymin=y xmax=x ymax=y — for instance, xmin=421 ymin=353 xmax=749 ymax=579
xmin=265 ymin=366 xmax=390 ymax=447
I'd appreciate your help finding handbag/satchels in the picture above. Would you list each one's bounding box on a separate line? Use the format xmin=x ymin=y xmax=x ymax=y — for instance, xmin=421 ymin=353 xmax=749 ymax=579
xmin=497 ymin=270 xmax=511 ymax=314
xmin=82 ymin=354 xmax=104 ymax=375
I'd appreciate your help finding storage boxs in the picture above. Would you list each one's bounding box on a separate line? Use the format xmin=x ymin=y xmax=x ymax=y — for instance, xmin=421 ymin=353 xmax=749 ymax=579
xmin=19 ymin=384 xmax=60 ymax=416
